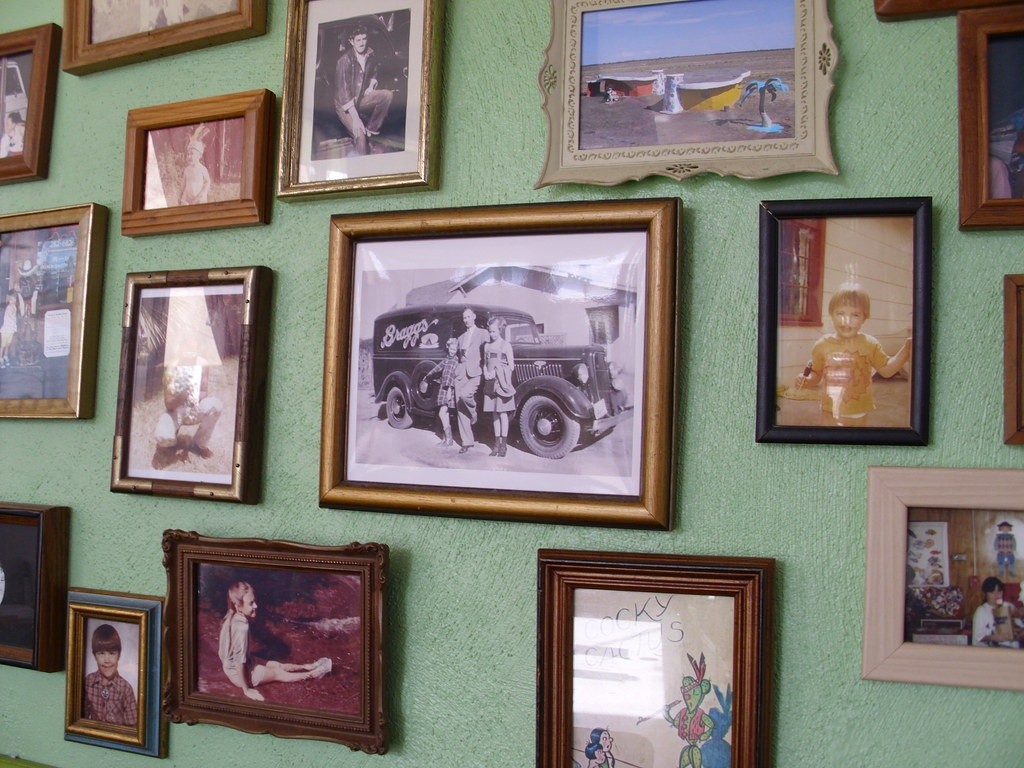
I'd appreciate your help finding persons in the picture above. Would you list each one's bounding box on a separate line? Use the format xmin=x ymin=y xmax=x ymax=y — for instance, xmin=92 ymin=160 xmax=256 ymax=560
xmin=80 ymin=623 xmax=139 ymax=729
xmin=793 ymin=282 xmax=915 ymax=431
xmin=3 ymin=112 xmax=28 ymax=154
xmin=1 ymin=288 xmax=19 ymax=370
xmin=216 ymin=580 xmax=333 ymax=704
xmin=332 ymin=24 xmax=392 ymax=158
xmin=152 ymin=335 xmax=224 ymax=464
xmin=420 ymin=306 xmax=517 ymax=455
xmin=970 ymin=577 xmax=1024 ymax=650
xmin=177 ymin=140 xmax=212 ymax=205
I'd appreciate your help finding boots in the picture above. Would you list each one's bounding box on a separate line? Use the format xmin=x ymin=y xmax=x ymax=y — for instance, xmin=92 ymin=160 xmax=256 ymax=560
xmin=437 ymin=429 xmax=453 ymax=447
xmin=489 ymin=437 xmax=507 ymax=457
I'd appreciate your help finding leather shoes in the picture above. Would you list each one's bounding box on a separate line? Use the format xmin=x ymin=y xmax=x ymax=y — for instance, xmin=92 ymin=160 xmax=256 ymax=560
xmin=459 ymin=446 xmax=470 ymax=454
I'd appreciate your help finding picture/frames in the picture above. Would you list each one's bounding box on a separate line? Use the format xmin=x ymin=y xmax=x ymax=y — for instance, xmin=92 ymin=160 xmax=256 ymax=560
xmin=0 ymin=502 xmax=71 ymax=672
xmin=862 ymin=466 xmax=1023 ymax=693
xmin=0 ymin=23 xmax=63 ymax=188
xmin=121 ymin=88 xmax=277 ymax=240
xmin=318 ymin=196 xmax=684 ymax=532
xmin=111 ymin=264 xmax=273 ymax=506
xmin=956 ymin=5 xmax=1024 ymax=231
xmin=755 ymin=196 xmax=930 ymax=447
xmin=61 ymin=1 xmax=265 ymax=78
xmin=160 ymin=528 xmax=390 ymax=756
xmin=0 ymin=202 xmax=111 ymax=421
xmin=276 ymin=1 xmax=442 ymax=203
xmin=535 ymin=547 xmax=775 ymax=768
xmin=62 ymin=585 xmax=169 ymax=760
xmin=533 ymin=1 xmax=840 ymax=189
xmin=1002 ymin=273 xmax=1024 ymax=446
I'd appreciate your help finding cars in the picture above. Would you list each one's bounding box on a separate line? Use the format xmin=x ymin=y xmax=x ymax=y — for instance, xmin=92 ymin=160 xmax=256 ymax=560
xmin=371 ymin=303 xmax=634 ymax=460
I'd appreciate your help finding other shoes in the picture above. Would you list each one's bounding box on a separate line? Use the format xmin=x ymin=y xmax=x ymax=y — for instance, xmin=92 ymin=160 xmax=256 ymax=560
xmin=200 ymin=448 xmax=212 ymax=459
xmin=316 ymin=657 xmax=332 ymax=680
xmin=0 ymin=355 xmax=11 ymax=369
xmin=178 ymin=435 xmax=192 ymax=450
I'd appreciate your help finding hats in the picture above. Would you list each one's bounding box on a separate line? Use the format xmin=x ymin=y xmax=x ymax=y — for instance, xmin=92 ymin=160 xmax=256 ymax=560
xmin=16 ymin=258 xmax=39 ymax=277
